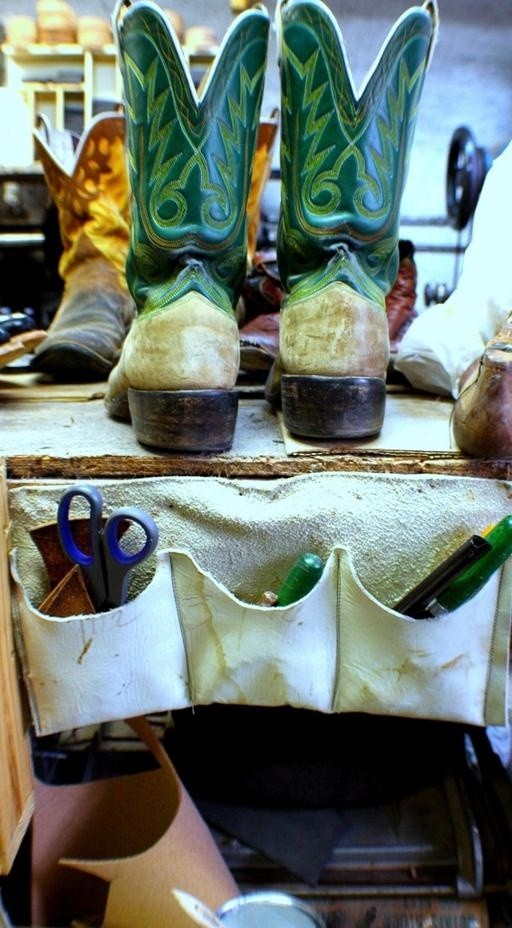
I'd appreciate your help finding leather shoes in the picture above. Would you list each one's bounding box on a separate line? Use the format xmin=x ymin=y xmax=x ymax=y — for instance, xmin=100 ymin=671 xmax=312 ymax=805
xmin=0 ymin=306 xmax=48 ymax=369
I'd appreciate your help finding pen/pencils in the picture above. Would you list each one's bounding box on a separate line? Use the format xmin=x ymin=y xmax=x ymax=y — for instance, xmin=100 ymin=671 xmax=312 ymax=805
xmin=258 ymin=552 xmax=325 ymax=609
xmin=393 ymin=514 xmax=512 ymax=621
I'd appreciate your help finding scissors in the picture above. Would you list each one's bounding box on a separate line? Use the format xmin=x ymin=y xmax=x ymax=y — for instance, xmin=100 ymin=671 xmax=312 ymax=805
xmin=54 ymin=486 xmax=158 ymax=615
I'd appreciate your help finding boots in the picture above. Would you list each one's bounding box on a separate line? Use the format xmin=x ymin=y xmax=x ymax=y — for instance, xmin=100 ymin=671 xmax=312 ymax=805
xmin=31 ymin=105 xmax=136 ymax=379
